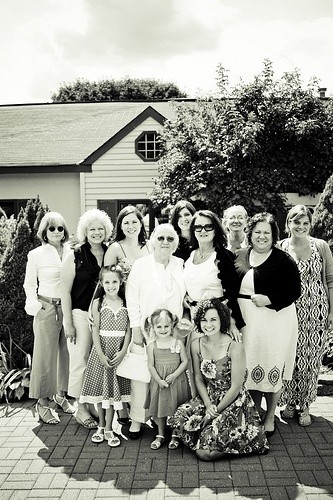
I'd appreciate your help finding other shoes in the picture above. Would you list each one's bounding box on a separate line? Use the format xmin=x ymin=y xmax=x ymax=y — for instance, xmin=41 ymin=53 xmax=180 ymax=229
xmin=263 ymin=416 xmax=276 ymax=437
xmin=117 ymin=410 xmax=129 ymax=424
xmin=127 ymin=422 xmax=143 ymax=441
xmin=88 ymin=410 xmax=100 ymax=423
xmin=282 ymin=404 xmax=296 ymax=418
xmin=299 ymin=408 xmax=311 ymax=427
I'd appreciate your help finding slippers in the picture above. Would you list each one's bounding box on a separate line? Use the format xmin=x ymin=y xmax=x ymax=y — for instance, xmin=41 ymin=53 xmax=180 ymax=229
xmin=76 ymin=415 xmax=98 ymax=428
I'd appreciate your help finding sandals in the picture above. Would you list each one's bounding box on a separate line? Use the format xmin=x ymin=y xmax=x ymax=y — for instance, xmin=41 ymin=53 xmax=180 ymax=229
xmin=168 ymin=434 xmax=180 ymax=450
xmin=150 ymin=434 xmax=165 ymax=449
xmin=103 ymin=428 xmax=121 ymax=447
xmin=91 ymin=425 xmax=105 ymax=443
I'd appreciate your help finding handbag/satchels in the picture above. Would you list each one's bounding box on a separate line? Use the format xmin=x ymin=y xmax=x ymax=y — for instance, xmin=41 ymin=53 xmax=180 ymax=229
xmin=115 ymin=340 xmax=153 ymax=384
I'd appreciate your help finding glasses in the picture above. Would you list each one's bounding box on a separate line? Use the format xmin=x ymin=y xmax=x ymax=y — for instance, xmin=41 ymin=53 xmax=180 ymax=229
xmin=46 ymin=226 xmax=65 ymax=233
xmin=157 ymin=236 xmax=175 ymax=242
xmin=101 ymin=264 xmax=117 ymax=272
xmin=226 ymin=214 xmax=245 ymax=225
xmin=192 ymin=223 xmax=216 ymax=232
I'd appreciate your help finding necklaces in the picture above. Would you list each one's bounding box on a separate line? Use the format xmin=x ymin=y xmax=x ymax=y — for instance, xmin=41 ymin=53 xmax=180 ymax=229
xmin=251 ymin=251 xmax=272 ymax=261
xmin=198 ymin=247 xmax=213 ymax=258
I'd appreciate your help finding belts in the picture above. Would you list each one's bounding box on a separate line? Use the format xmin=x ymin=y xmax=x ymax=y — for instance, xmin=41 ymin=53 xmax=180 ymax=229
xmin=238 ymin=293 xmax=251 ymax=300
xmin=187 ymin=295 xmax=227 ymax=306
xmin=38 ymin=294 xmax=61 ymax=322
xmin=100 ymin=330 xmax=126 ymax=338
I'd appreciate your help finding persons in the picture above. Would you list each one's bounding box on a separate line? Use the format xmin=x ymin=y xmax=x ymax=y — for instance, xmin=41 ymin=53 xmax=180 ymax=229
xmin=275 ymin=205 xmax=333 ymax=426
xmin=125 ymin=223 xmax=189 ymax=440
xmin=145 ymin=309 xmax=188 ymax=450
xmin=23 ymin=212 xmax=75 ymax=424
xmin=183 ymin=210 xmax=238 ymax=397
xmin=136 ymin=204 xmax=159 ymax=238
xmin=222 ymin=205 xmax=251 ymax=257
xmin=171 ymin=299 xmax=270 ymax=461
xmin=79 ymin=264 xmax=130 ymax=447
xmin=59 ymin=209 xmax=113 ymax=429
xmin=170 ymin=200 xmax=197 ymax=267
xmin=224 ymin=212 xmax=301 ymax=437
xmin=104 ymin=206 xmax=154 ymax=307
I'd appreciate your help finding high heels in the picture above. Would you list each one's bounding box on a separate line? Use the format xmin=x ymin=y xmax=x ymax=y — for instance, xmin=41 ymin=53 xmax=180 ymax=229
xmin=55 ymin=394 xmax=76 ymax=414
xmin=36 ymin=400 xmax=59 ymax=425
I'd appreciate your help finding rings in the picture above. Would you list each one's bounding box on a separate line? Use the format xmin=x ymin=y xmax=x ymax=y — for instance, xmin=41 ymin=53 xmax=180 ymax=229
xmin=73 ymin=338 xmax=75 ymax=340
xmin=206 ymin=414 xmax=211 ymax=420
xmin=240 ymin=333 xmax=242 ymax=336
xmin=69 ymin=338 xmax=72 ymax=340
